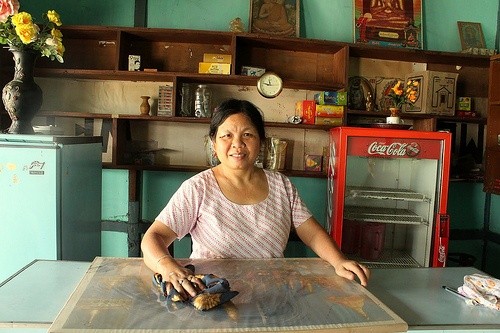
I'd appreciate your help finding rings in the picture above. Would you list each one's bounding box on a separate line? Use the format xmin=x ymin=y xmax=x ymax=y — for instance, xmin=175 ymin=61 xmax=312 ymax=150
xmin=179 ymin=277 xmax=187 ymax=285
xmin=188 ymin=275 xmax=196 ymax=282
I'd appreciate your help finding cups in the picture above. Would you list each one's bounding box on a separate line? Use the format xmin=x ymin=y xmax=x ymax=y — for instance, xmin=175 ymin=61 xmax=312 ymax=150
xmin=386 ymin=117 xmax=404 ymax=124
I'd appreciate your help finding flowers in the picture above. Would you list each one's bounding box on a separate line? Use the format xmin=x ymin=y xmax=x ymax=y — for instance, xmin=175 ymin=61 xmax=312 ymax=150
xmin=0 ymin=0 xmax=65 ymax=63
xmin=388 ymin=80 xmax=419 ymax=112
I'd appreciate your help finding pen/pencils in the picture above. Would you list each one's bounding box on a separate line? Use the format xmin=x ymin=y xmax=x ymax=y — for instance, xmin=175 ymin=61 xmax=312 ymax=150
xmin=442 ymin=286 xmax=479 ymax=304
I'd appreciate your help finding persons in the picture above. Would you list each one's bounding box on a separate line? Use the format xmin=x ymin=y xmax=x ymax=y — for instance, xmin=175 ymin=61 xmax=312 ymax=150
xmin=139 ymin=97 xmax=372 ymax=300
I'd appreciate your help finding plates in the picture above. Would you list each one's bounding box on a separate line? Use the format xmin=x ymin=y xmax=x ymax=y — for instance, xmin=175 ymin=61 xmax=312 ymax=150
xmin=376 ymin=123 xmax=413 ymax=130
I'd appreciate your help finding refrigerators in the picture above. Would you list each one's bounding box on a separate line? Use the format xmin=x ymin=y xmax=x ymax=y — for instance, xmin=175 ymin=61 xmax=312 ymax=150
xmin=325 ymin=126 xmax=452 ymax=268
xmin=0 ymin=132 xmax=103 ymax=283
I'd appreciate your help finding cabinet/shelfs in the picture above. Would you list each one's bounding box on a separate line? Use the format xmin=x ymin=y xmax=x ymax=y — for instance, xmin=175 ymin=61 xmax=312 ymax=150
xmin=0 ymin=24 xmax=490 ymax=182
xmin=483 ymin=54 xmax=500 ymax=195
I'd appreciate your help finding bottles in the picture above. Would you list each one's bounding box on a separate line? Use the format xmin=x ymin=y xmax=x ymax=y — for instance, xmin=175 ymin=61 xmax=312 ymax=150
xmin=139 ymin=96 xmax=151 ymax=116
xmin=180 ymin=83 xmax=214 ymax=119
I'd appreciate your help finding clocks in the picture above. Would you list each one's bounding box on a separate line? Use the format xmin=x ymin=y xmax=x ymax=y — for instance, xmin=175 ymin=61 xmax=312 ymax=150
xmin=257 ymin=71 xmax=283 ymax=99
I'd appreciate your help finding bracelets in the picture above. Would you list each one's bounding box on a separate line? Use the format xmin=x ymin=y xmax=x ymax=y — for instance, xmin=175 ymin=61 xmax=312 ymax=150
xmin=153 ymin=255 xmax=175 ymax=261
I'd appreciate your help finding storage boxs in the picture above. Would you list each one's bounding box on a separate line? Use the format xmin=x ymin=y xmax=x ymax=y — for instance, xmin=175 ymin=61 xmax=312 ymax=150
xmin=405 ymin=71 xmax=459 ymax=116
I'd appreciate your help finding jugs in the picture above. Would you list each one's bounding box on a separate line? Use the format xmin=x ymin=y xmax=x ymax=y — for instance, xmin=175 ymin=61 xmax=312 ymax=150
xmin=342 ymin=221 xmax=385 ymax=261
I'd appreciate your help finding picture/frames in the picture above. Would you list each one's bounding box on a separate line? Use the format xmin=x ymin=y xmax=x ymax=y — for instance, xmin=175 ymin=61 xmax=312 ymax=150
xmin=248 ymin=0 xmax=300 ymax=38
xmin=457 ymin=21 xmax=486 ymax=50
xmin=353 ymin=0 xmax=423 ymax=50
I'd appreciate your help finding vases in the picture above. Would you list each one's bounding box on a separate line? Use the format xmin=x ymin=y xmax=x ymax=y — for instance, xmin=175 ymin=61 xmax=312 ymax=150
xmin=389 ymin=107 xmax=401 ymax=117
xmin=2 ymin=48 xmax=43 ymax=135
